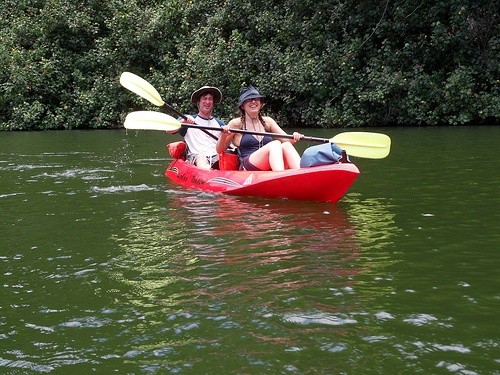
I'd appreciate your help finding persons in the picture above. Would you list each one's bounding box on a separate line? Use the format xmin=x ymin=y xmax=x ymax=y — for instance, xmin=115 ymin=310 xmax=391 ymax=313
xmin=216 ymin=86 xmax=304 ymax=172
xmin=165 ymin=85 xmax=237 ymax=170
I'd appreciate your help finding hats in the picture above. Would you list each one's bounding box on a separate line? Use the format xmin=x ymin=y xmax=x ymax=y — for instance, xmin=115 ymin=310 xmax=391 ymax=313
xmin=238 ymin=84 xmax=266 ymax=110
xmin=190 ymin=86 xmax=222 ymax=104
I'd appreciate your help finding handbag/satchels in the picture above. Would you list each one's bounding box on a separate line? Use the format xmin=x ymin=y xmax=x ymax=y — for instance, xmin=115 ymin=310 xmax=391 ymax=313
xmin=300 ymin=142 xmax=351 ymax=168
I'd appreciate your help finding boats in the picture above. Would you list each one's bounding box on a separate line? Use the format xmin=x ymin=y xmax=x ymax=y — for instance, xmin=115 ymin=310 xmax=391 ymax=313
xmin=165 ymin=141 xmax=360 ymax=203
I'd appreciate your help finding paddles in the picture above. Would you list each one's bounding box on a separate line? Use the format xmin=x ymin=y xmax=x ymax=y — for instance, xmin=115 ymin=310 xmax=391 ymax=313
xmin=119 ymin=71 xmax=235 ymax=151
xmin=121 ymin=109 xmax=392 ymax=161
